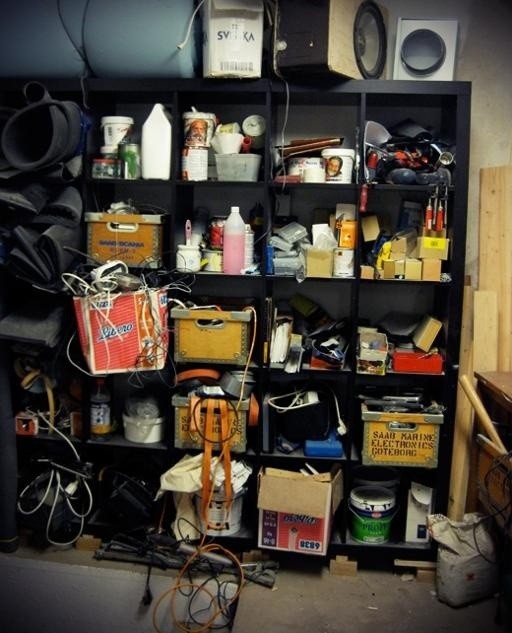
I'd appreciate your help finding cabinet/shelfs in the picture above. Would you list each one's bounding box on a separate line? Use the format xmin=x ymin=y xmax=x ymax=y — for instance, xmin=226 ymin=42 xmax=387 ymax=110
xmin=0 ymin=75 xmax=473 ymax=567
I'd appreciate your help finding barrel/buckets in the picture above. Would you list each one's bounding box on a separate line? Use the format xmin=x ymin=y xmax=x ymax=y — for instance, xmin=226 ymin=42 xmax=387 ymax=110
xmin=353 ymin=464 xmax=401 ymax=494
xmin=140 ymin=102 xmax=176 ymax=181
xmin=193 ymin=486 xmax=247 ymax=536
xmin=347 ymin=486 xmax=401 ymax=546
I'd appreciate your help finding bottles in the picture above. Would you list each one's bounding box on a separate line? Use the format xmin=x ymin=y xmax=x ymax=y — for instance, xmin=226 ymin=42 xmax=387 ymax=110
xmin=223 ymin=206 xmax=246 ymax=275
xmin=90 ymin=377 xmax=112 ymax=440
xmin=176 ymin=245 xmax=201 ymax=273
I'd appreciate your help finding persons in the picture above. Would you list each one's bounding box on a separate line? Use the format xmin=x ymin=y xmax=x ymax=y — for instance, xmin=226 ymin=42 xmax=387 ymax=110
xmin=326 ymin=157 xmax=342 ymax=177
xmin=185 ymin=119 xmax=207 ymax=141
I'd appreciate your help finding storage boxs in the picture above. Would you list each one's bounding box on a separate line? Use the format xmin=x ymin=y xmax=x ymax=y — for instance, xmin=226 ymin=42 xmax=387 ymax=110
xmin=170 ymin=393 xmax=249 ymax=454
xmin=253 ymin=461 xmax=344 ymax=558
xmin=81 ymin=208 xmax=169 ymax=268
xmin=358 ymin=397 xmax=444 ymax=469
xmin=69 ymin=289 xmax=256 ymax=376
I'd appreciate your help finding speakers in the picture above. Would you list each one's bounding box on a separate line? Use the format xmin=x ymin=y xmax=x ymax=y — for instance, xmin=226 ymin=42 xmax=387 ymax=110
xmin=274 ymin=0 xmax=389 ymax=82
xmin=393 ymin=16 xmax=460 ymax=81
